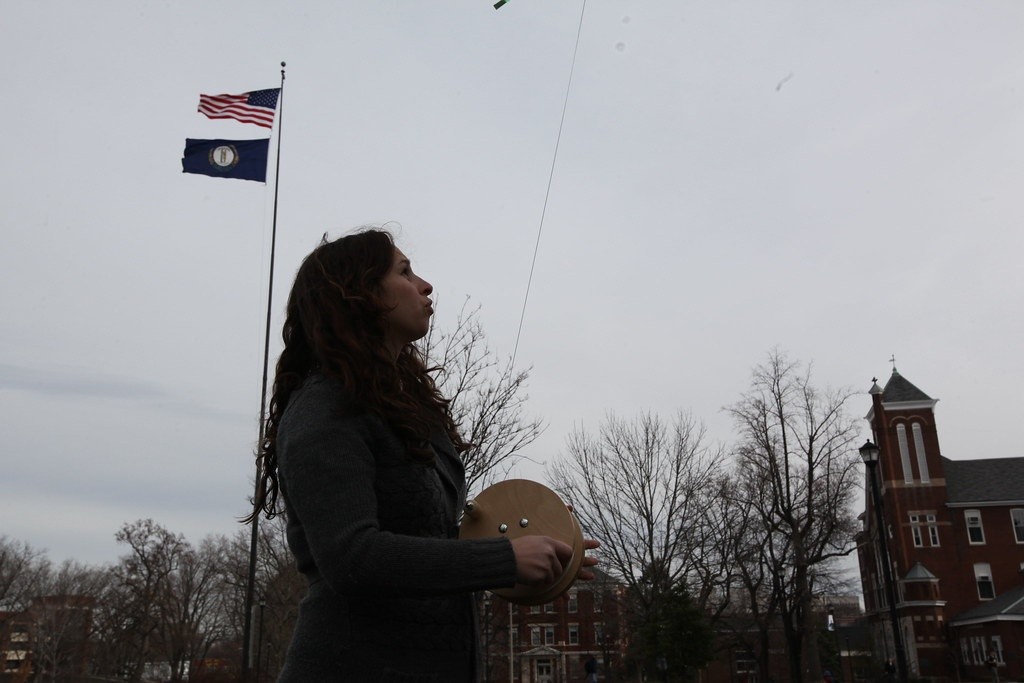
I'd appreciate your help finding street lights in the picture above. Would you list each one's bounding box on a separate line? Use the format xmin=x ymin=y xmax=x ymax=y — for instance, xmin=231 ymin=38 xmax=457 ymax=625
xmin=844 ymin=633 xmax=854 ymax=683
xmin=858 ymin=437 xmax=911 ymax=682
xmin=257 ymin=596 xmax=267 ymax=681
xmin=828 ymin=604 xmax=845 ymax=683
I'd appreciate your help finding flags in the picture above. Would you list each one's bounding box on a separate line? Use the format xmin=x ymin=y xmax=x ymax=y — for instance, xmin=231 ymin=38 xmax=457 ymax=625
xmin=197 ymin=88 xmax=281 ymax=129
xmin=182 ymin=138 xmax=270 ymax=183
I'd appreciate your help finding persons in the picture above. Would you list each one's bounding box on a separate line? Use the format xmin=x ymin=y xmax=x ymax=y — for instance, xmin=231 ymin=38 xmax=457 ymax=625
xmin=240 ymin=229 xmax=600 ymax=683
